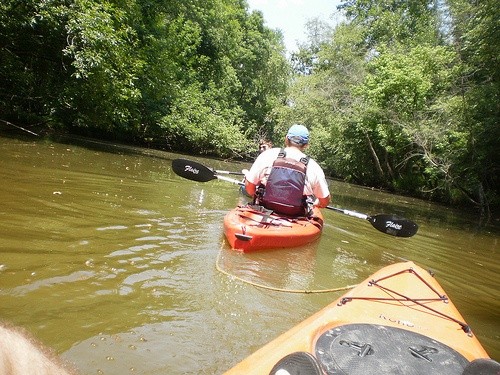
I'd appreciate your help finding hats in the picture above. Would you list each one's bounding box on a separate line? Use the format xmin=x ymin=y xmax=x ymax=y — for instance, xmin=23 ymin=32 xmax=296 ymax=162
xmin=287 ymin=124 xmax=309 ymax=144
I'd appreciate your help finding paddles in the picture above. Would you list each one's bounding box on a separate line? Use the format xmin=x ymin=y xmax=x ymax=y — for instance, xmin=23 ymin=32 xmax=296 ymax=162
xmin=171 ymin=158 xmax=419 ymax=238
xmin=204 ymin=167 xmax=247 ymax=174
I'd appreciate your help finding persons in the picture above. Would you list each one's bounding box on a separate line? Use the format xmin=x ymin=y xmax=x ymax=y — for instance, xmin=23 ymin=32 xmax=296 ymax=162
xmin=242 ymin=122 xmax=332 ymax=217
xmin=259 ymin=139 xmax=273 ymax=154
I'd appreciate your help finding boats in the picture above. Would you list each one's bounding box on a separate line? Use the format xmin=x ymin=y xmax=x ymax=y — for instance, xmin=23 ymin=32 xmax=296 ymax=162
xmin=224 ymin=199 xmax=324 ymax=253
